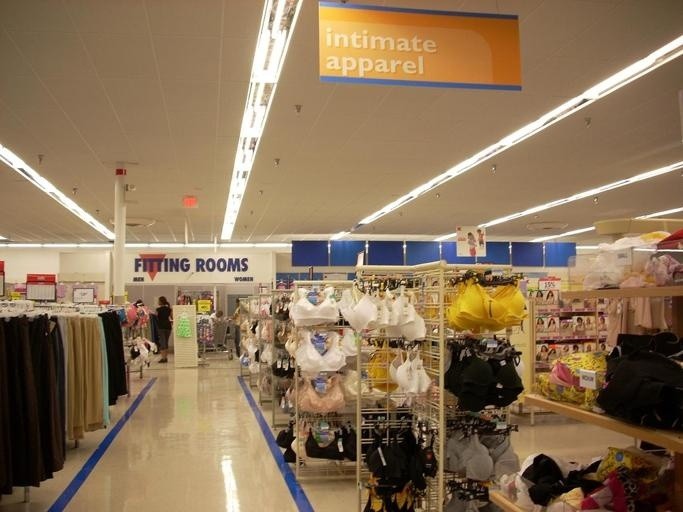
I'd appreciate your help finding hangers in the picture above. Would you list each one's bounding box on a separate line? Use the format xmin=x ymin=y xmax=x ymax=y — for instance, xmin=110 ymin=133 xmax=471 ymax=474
xmin=3 ymin=297 xmax=117 ymax=324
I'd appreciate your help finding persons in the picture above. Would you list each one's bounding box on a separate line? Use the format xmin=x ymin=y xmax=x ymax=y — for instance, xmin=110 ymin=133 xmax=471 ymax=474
xmin=230 ymin=298 xmax=239 ymax=358
xmin=477 ymin=230 xmax=484 ymax=247
xmin=210 ymin=311 xmax=223 ymax=321
xmin=534 ymin=290 xmax=606 ymax=361
xmin=466 ymin=231 xmax=477 ymax=256
xmin=148 ymin=295 xmax=173 ymax=363
